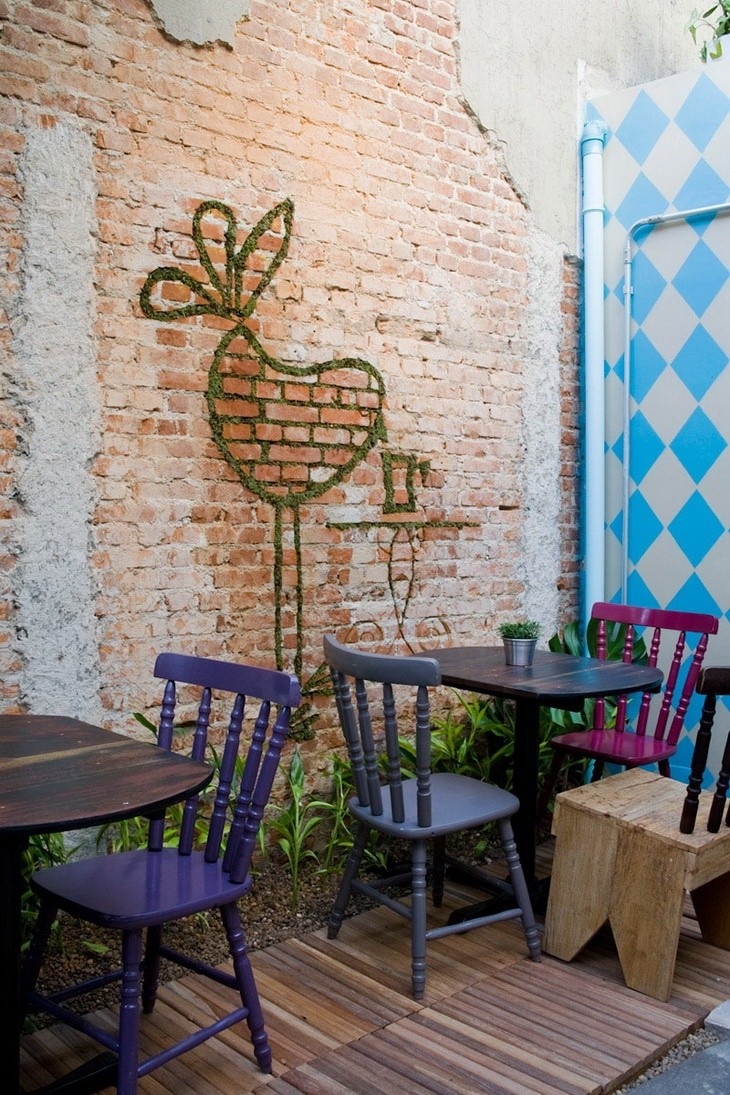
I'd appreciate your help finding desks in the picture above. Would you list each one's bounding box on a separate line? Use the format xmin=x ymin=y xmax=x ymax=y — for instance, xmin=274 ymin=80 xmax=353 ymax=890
xmin=0 ymin=714 xmax=215 ymax=1095
xmin=401 ymin=645 xmax=664 ymax=933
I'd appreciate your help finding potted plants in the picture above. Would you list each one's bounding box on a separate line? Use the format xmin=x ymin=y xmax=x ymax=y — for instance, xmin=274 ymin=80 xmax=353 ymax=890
xmin=499 ymin=620 xmax=544 ymax=666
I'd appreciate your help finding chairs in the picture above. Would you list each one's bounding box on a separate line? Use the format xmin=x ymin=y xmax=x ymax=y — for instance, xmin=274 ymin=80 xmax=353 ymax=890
xmin=322 ymin=633 xmax=541 ymax=1000
xmin=541 ymin=664 xmax=730 ymax=1002
xmin=17 ymin=652 xmax=301 ymax=1095
xmin=550 ymin=602 xmax=720 ymax=785
